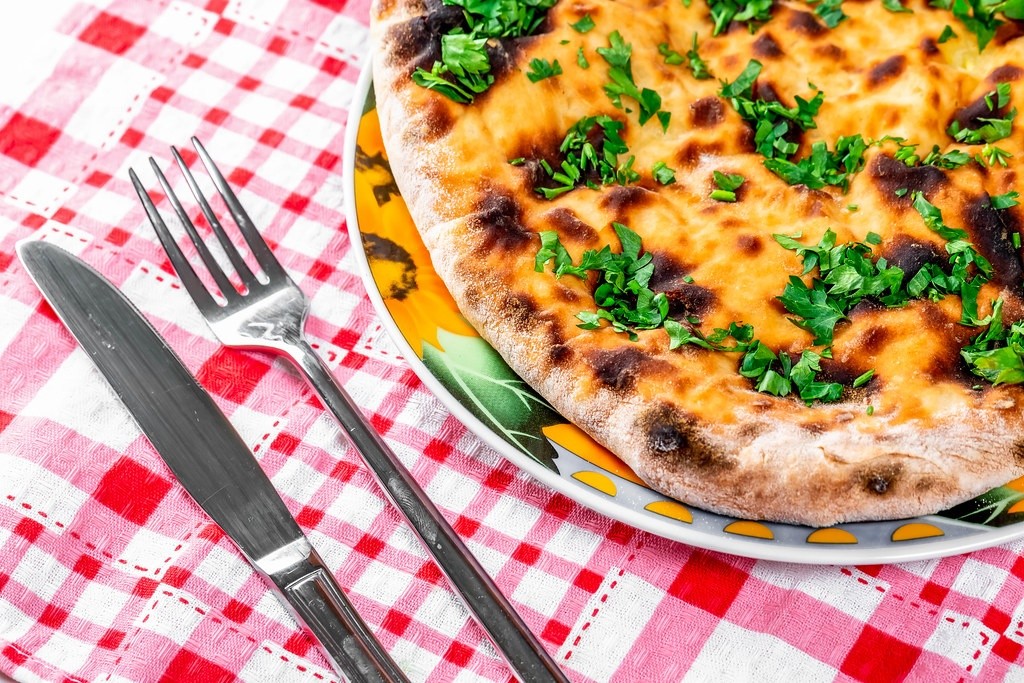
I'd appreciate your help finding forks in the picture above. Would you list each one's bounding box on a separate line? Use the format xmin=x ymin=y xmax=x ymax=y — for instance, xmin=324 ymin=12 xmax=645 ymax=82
xmin=126 ymin=136 xmax=573 ymax=683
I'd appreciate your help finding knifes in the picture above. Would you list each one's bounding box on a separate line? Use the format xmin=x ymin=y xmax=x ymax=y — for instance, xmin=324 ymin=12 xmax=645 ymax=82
xmin=14 ymin=238 xmax=410 ymax=683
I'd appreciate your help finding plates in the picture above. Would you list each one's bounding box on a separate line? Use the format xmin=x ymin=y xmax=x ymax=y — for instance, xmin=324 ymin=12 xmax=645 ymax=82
xmin=344 ymin=58 xmax=1024 ymax=566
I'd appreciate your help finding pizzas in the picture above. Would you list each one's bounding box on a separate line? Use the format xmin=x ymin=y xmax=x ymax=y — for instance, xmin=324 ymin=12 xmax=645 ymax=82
xmin=365 ymin=0 xmax=1024 ymax=523
